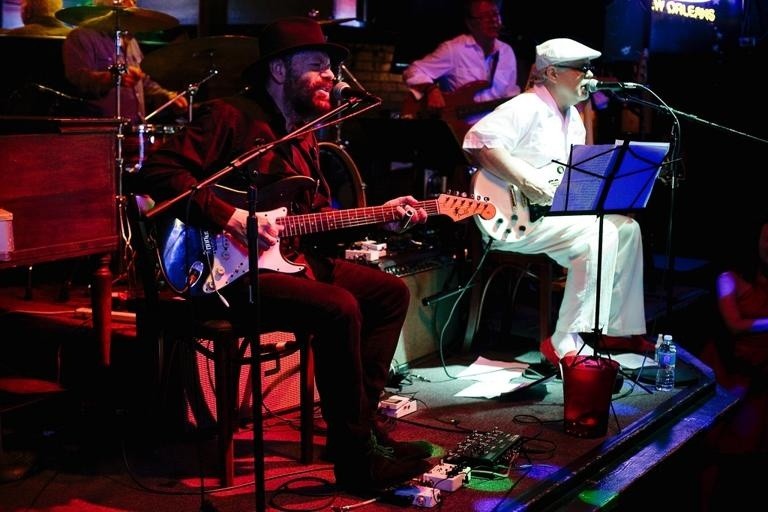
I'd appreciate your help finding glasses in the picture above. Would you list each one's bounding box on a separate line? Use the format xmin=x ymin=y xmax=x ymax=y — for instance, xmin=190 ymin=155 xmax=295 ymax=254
xmin=472 ymin=9 xmax=500 ymax=19
xmin=551 ymin=63 xmax=596 ymax=73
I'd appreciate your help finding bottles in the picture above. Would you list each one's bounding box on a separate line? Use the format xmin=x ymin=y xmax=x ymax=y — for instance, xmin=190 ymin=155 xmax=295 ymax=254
xmin=655 ymin=334 xmax=676 ymax=387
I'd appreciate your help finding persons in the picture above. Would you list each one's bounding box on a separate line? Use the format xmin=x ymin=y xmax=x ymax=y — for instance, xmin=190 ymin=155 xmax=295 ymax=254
xmin=124 ymin=15 xmax=433 ymax=490
xmin=713 ymin=211 xmax=766 ymax=374
xmin=62 ymin=1 xmax=190 ymax=167
xmin=403 ymin=0 xmax=523 ymax=132
xmin=0 ymin=0 xmax=75 ymax=39
xmin=458 ymin=33 xmax=659 ymax=390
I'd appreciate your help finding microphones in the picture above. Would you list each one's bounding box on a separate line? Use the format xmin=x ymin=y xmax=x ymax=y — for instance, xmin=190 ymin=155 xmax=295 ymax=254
xmin=332 ymin=81 xmax=375 ymax=104
xmin=588 ymin=78 xmax=636 ymax=95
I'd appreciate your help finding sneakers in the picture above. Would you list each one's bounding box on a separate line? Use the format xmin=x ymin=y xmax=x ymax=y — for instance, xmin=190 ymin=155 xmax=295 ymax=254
xmin=371 ymin=427 xmax=432 ymax=462
xmin=333 ymin=444 xmax=432 ymax=496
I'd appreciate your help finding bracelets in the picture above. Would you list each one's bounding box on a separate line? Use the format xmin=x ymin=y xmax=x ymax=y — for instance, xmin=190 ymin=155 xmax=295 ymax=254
xmin=422 ymin=83 xmax=438 ymax=93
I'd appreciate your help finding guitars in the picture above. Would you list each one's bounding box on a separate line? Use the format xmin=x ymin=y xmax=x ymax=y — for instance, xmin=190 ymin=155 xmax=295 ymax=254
xmin=154 ymin=175 xmax=498 ymax=295
xmin=400 ymin=78 xmax=525 ymax=142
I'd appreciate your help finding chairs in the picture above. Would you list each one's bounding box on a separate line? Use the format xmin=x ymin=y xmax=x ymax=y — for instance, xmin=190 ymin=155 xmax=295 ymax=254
xmin=126 ymin=195 xmax=318 ymax=485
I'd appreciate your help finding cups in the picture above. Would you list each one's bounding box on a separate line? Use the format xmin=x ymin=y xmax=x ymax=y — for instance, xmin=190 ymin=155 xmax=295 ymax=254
xmin=562 ymin=355 xmax=620 ymax=436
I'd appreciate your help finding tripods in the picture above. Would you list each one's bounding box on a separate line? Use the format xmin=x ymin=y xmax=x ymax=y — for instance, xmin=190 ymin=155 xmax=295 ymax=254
xmin=502 ymin=216 xmax=653 ymax=435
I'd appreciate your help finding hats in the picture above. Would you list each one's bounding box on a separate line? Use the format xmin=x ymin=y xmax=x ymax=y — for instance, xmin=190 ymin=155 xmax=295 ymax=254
xmin=535 ymin=38 xmax=602 ymax=72
xmin=240 ymin=15 xmax=351 ymax=89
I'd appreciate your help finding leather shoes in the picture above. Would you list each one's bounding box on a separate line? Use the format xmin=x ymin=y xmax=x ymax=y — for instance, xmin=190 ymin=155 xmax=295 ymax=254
xmin=539 ymin=335 xmax=561 ymax=369
xmin=598 ymin=336 xmax=656 ymax=354
